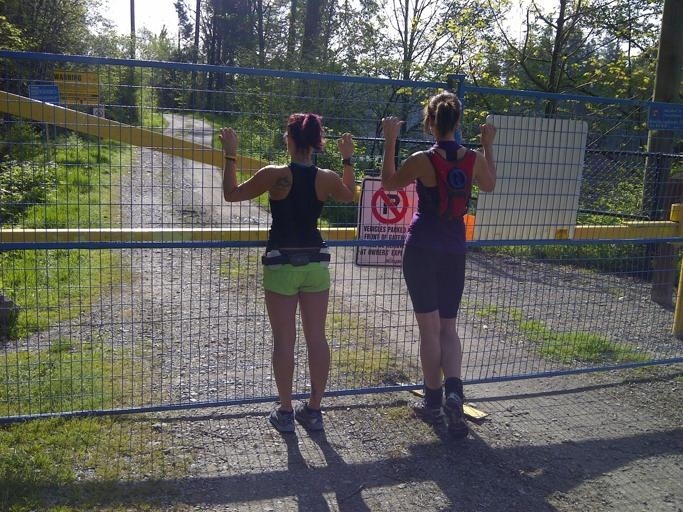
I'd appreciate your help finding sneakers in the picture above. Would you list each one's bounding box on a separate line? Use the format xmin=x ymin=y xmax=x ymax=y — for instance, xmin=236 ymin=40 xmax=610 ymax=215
xmin=442 ymin=390 xmax=470 ymax=443
xmin=295 ymin=401 xmax=324 ymax=434
xmin=411 ymin=400 xmax=445 ymax=427
xmin=269 ymin=406 xmax=297 ymax=434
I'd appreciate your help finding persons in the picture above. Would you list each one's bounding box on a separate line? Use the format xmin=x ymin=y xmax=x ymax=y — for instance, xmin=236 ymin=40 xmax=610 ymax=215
xmin=378 ymin=91 xmax=498 ymax=440
xmin=216 ymin=112 xmax=357 ymax=433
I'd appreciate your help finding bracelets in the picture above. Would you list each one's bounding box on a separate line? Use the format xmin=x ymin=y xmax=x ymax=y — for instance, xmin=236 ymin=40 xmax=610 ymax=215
xmin=222 ymin=154 xmax=236 ymax=161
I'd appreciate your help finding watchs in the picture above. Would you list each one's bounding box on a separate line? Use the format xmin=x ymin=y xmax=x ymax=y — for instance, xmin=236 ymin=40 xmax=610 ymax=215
xmin=340 ymin=158 xmax=355 ymax=165
